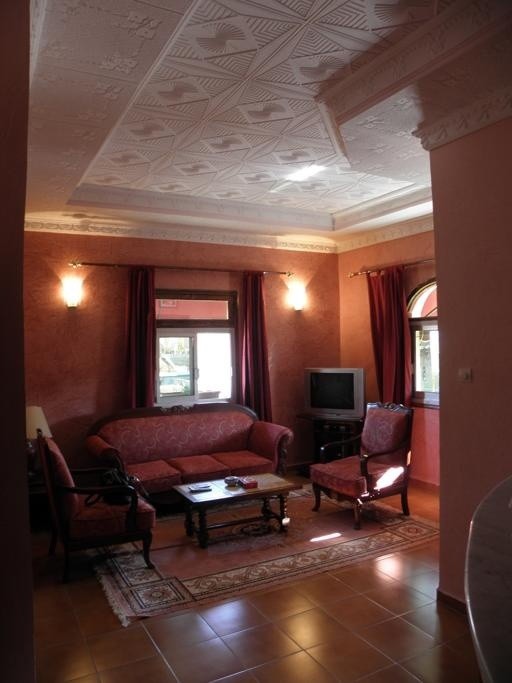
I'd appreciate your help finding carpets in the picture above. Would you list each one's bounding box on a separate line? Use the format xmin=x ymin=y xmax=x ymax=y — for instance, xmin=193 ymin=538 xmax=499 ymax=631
xmin=81 ymin=482 xmax=440 ymax=628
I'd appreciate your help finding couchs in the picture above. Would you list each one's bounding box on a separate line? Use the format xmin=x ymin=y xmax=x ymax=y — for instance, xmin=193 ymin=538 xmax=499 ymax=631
xmin=83 ymin=403 xmax=293 ymax=517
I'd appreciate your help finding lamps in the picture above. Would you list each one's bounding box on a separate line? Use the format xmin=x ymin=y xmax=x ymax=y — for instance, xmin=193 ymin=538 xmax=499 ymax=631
xmin=26 ymin=406 xmax=54 ymax=494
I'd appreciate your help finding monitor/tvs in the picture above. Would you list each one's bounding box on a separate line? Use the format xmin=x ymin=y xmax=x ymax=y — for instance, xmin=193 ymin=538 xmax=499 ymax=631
xmin=303 ymin=367 xmax=365 ymax=422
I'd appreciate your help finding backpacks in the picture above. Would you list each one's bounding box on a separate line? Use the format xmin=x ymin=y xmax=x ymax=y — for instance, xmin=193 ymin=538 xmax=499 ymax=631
xmin=85 ymin=448 xmax=137 ymax=507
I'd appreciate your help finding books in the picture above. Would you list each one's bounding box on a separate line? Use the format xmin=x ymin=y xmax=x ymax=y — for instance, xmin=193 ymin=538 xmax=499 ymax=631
xmin=187 ymin=480 xmax=214 ymax=492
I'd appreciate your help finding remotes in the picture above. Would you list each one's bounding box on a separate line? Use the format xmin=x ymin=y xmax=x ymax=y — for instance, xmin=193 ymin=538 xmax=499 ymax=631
xmin=188 ymin=483 xmax=212 ymax=493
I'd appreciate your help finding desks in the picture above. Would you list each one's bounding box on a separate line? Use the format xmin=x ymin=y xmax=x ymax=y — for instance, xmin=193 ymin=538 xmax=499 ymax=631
xmin=297 ymin=414 xmax=364 ymax=462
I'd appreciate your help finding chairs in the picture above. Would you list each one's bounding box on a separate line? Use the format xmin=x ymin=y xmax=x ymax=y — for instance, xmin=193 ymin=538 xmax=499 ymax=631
xmin=310 ymin=401 xmax=415 ymax=529
xmin=34 ymin=428 xmax=156 ymax=581
